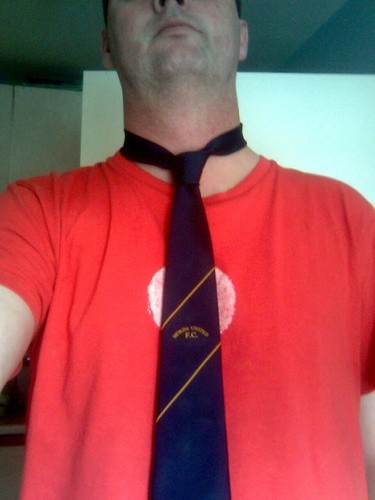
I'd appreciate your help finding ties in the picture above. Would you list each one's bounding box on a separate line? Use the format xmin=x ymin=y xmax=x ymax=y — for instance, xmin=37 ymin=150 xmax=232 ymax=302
xmin=120 ymin=124 xmax=248 ymax=499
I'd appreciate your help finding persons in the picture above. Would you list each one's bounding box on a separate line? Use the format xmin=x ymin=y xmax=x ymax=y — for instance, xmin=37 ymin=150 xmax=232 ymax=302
xmin=0 ymin=0 xmax=374 ymax=500
